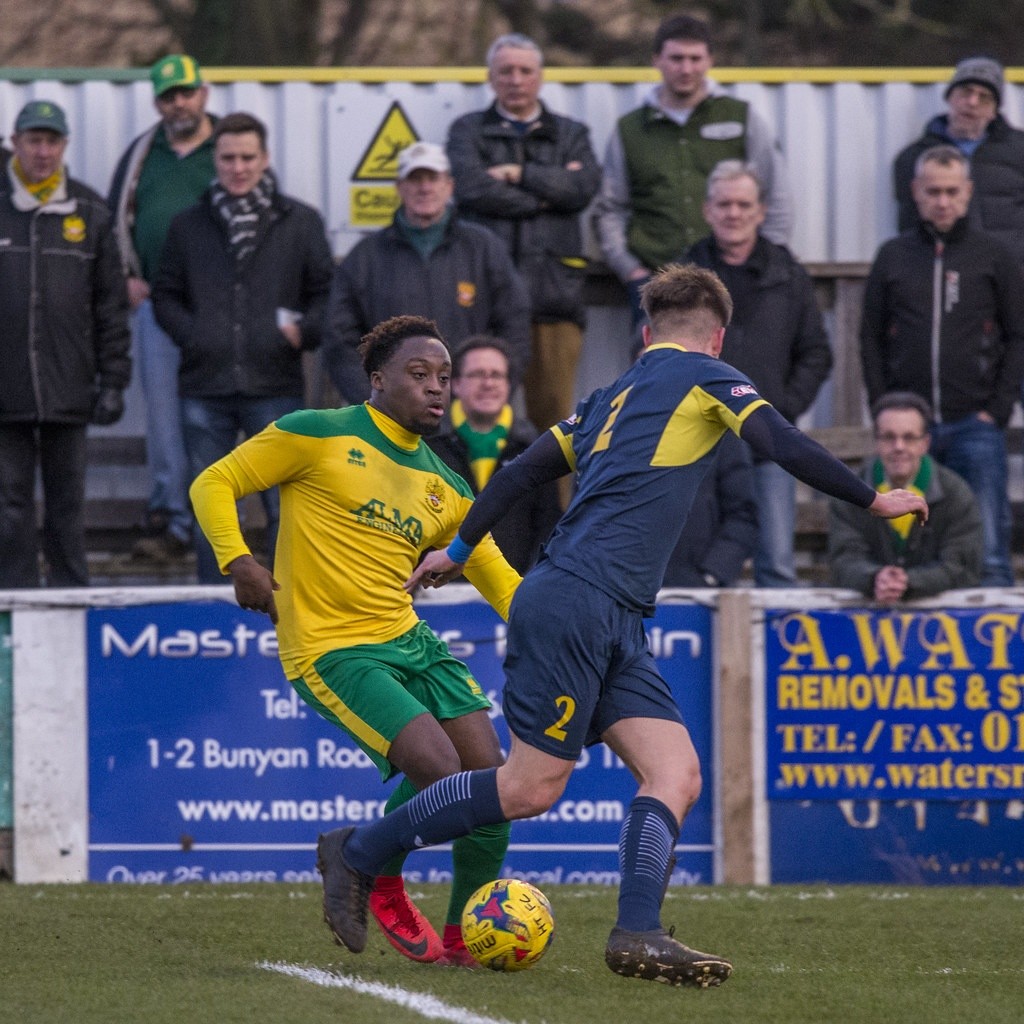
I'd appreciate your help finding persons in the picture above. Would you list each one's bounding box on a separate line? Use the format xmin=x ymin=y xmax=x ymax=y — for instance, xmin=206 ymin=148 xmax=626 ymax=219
xmin=317 ymin=260 xmax=931 ymax=994
xmin=441 ymin=34 xmax=600 ymax=436
xmin=103 ymin=52 xmax=229 ymax=566
xmin=887 ymin=56 xmax=1024 ymax=267
xmin=661 ymin=429 xmax=762 ymax=589
xmin=189 ymin=314 xmax=527 ymax=967
xmin=317 ymin=139 xmax=533 ymax=410
xmin=0 ymin=101 xmax=135 ymax=592
xmin=418 ymin=332 xmax=569 ymax=582
xmin=683 ymin=158 xmax=848 ymax=587
xmin=822 ymin=394 xmax=984 ymax=604
xmin=592 ymin=19 xmax=795 ymax=366
xmin=151 ymin=114 xmax=335 ymax=583
xmin=856 ymin=146 xmax=1023 ymax=586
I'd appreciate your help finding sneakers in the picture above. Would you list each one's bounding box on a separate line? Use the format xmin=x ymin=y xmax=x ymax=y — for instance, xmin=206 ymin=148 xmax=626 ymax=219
xmin=316 ymin=825 xmax=368 ymax=953
xmin=605 ymin=926 xmax=731 ymax=989
xmin=367 ymin=877 xmax=443 ymax=962
xmin=435 ymin=925 xmax=481 ymax=966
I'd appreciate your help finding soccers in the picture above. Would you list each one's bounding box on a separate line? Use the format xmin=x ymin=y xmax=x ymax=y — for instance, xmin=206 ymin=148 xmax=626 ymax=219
xmin=460 ymin=878 xmax=555 ymax=973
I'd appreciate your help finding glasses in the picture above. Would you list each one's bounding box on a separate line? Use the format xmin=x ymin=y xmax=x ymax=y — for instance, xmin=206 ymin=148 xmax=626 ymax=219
xmin=874 ymin=433 xmax=926 ymax=445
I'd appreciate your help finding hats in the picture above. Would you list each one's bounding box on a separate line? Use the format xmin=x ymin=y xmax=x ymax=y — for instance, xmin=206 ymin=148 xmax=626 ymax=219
xmin=946 ymin=49 xmax=1002 ymax=105
xmin=151 ymin=54 xmax=200 ymax=96
xmin=15 ymin=101 xmax=67 ymax=134
xmin=399 ymin=144 xmax=450 ymax=180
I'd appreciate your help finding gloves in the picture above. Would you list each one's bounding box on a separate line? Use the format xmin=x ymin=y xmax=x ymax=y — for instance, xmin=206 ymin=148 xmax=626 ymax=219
xmin=92 ymin=387 xmax=123 ymax=424
